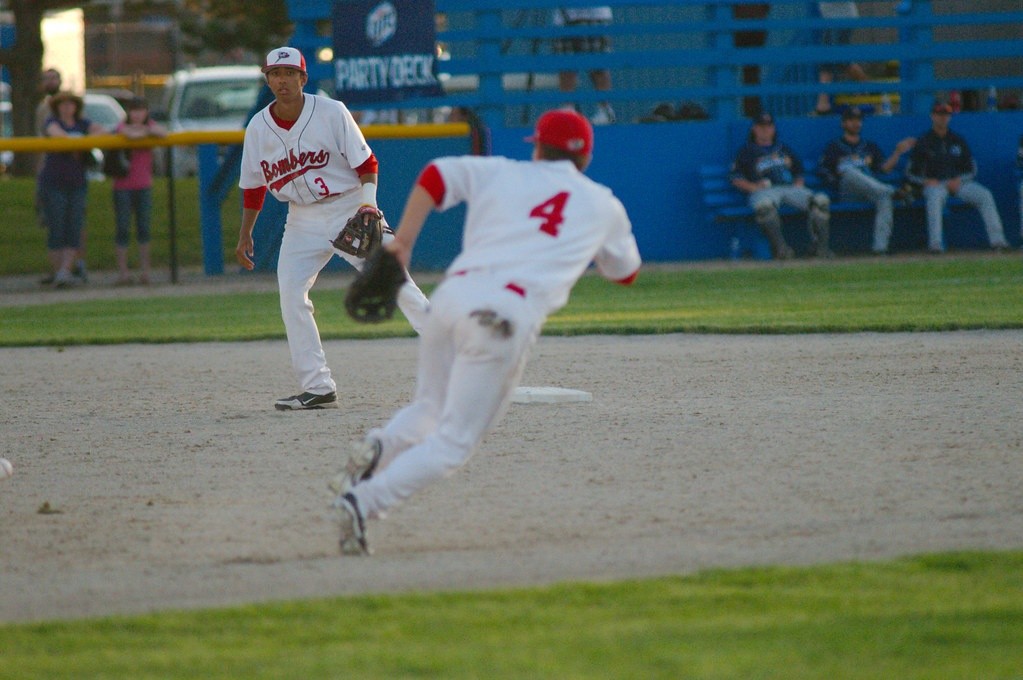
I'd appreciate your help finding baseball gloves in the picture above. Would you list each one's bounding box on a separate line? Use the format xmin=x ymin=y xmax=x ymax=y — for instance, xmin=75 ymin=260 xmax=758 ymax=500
xmin=344 ymin=246 xmax=407 ymax=325
xmin=332 ymin=204 xmax=384 ymax=260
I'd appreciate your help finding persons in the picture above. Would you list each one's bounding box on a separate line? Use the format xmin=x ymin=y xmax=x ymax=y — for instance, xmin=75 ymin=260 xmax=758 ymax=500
xmin=814 ymin=0 xmax=876 ymax=112
xmin=116 ymin=95 xmax=168 ymax=286
xmin=330 ymin=108 xmax=643 ymax=557
xmin=34 ymin=89 xmax=112 ymax=290
xmin=825 ymin=102 xmax=915 ymax=256
xmin=731 ymin=111 xmax=837 ymax=258
xmin=235 ymin=47 xmax=431 ymax=409
xmin=905 ymin=100 xmax=1010 ymax=255
xmin=550 ymin=5 xmax=617 ymax=125
xmin=31 ymin=70 xmax=86 ymax=286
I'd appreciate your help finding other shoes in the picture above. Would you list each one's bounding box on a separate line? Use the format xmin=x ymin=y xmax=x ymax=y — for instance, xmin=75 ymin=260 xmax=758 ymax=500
xmin=590 ymin=103 xmax=618 ymax=127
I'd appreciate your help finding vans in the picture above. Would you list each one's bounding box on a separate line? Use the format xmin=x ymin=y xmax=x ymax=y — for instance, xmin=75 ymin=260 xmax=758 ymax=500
xmin=141 ymin=60 xmax=266 ymax=176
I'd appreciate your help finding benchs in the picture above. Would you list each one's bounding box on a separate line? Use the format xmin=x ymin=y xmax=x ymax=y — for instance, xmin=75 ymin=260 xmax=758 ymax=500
xmin=702 ymin=164 xmax=969 ymax=259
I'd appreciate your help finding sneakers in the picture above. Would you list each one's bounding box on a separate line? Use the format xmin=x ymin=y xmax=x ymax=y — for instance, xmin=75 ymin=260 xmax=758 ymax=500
xmin=334 ymin=492 xmax=373 ymax=559
xmin=327 ymin=435 xmax=382 ymax=508
xmin=274 ymin=391 xmax=339 ymax=409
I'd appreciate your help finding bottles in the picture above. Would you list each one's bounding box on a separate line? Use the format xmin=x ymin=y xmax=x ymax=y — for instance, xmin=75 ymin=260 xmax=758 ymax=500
xmin=988 ymin=85 xmax=997 ymax=111
xmin=881 ymin=92 xmax=892 ymax=116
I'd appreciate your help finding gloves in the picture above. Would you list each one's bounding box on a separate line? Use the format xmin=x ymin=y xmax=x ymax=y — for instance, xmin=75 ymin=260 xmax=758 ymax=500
xmin=470 ymin=309 xmax=514 ymax=337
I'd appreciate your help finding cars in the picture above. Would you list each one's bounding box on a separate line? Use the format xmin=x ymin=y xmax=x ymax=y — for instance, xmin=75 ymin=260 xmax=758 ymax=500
xmin=81 ymin=95 xmax=128 ymax=135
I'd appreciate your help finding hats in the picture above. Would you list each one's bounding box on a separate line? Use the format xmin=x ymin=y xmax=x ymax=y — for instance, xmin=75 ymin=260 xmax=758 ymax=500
xmin=934 ymin=102 xmax=953 ymax=115
xmin=48 ymin=91 xmax=83 ymax=118
xmin=755 ymin=111 xmax=776 ymax=125
xmin=524 ymin=109 xmax=593 ymax=154
xmin=845 ymin=106 xmax=863 ymax=118
xmin=261 ymin=47 xmax=306 ymax=74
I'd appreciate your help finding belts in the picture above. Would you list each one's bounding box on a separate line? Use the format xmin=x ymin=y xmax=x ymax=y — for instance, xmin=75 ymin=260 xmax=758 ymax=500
xmin=455 ymin=271 xmax=528 ymax=298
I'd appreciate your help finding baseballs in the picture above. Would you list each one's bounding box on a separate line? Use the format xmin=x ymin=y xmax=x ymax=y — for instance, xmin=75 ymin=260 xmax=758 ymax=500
xmin=0 ymin=458 xmax=13 ymax=481
